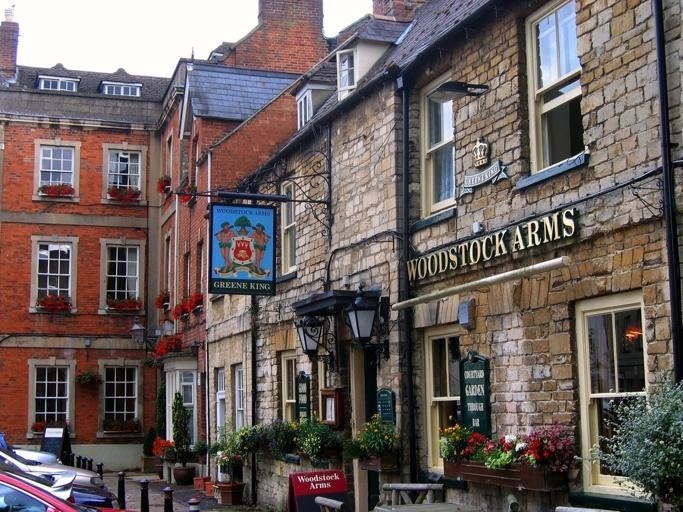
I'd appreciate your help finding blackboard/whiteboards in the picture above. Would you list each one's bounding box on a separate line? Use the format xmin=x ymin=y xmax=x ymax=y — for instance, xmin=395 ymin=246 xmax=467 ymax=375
xmin=459 ymin=355 xmax=490 ymax=437
xmin=295 ymin=371 xmax=311 ymax=425
xmin=376 ymin=388 xmax=396 ymax=426
xmin=295 ymin=492 xmax=352 ymax=512
xmin=40 ymin=437 xmax=63 ymax=461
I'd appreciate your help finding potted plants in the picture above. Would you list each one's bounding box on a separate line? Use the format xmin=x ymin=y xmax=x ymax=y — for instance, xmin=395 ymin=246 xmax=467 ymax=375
xmin=141 ymin=426 xmax=156 ymax=474
xmin=153 ymin=437 xmax=175 ymax=479
xmin=171 ymin=391 xmax=197 ymax=486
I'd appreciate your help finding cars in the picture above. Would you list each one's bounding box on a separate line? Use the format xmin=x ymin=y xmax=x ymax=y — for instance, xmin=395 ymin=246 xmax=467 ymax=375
xmin=0 ymin=447 xmax=138 ymax=512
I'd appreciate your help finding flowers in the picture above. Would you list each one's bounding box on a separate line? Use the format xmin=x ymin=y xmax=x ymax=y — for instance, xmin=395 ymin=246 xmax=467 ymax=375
xmin=354 ymin=413 xmax=401 ymax=457
xmin=214 ymin=450 xmax=246 ymax=482
xmin=436 ymin=422 xmax=576 ymax=474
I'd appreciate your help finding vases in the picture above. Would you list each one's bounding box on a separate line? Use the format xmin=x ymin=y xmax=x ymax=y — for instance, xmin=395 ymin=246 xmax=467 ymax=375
xmin=203 ymin=481 xmax=216 ymax=497
xmin=368 ymin=454 xmax=398 ymax=470
xmin=192 ymin=476 xmax=211 ymax=492
xmin=442 ymin=457 xmax=570 ymax=490
xmin=217 ymin=481 xmax=247 ymax=507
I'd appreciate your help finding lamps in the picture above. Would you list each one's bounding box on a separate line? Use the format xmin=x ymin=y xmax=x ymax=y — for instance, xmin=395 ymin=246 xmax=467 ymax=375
xmin=341 ymin=284 xmax=390 ymax=361
xmin=427 ymin=79 xmax=489 ymax=104
xmin=293 ymin=313 xmax=335 ymax=373
xmin=128 ymin=317 xmax=157 ymax=351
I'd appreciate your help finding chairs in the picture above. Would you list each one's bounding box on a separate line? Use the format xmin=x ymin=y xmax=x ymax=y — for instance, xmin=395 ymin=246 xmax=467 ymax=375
xmin=315 ymin=495 xmax=344 ymax=511
xmin=374 ymin=482 xmax=620 ymax=512
